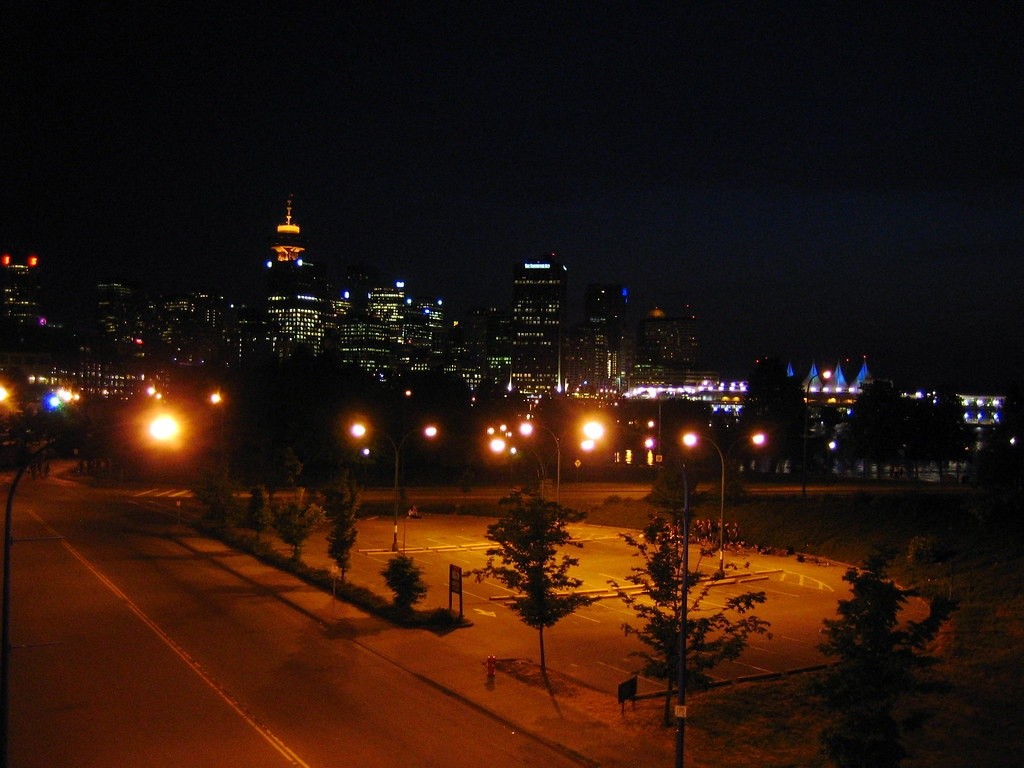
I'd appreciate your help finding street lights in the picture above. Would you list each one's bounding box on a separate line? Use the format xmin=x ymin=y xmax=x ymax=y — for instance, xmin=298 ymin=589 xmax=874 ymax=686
xmin=490 ymin=436 xmax=596 ymax=501
xmin=646 ymin=386 xmax=662 ymax=473
xmin=801 ymin=369 xmax=833 ymax=495
xmin=208 ymin=392 xmax=226 ymax=490
xmin=681 ymin=427 xmax=768 ymax=580
xmin=0 ymin=406 xmax=187 ymax=768
xmin=518 ymin=415 xmax=604 ymax=521
xmin=349 ymin=422 xmax=438 ymax=554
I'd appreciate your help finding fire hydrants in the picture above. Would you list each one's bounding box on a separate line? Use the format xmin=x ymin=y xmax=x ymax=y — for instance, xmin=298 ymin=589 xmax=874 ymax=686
xmin=486 ymin=654 xmax=496 ymax=678
